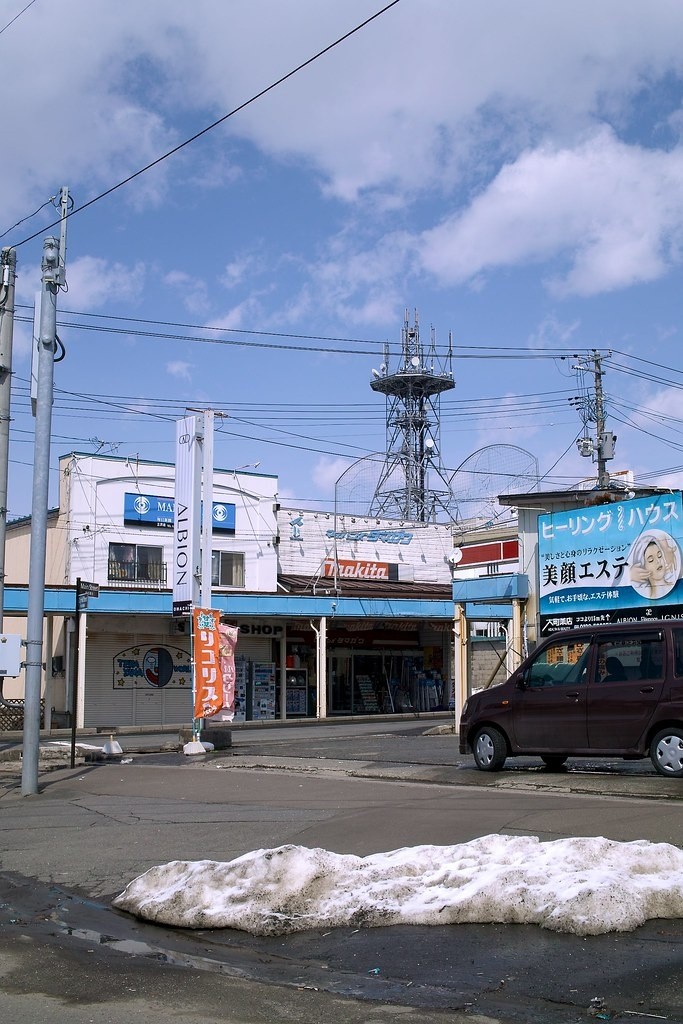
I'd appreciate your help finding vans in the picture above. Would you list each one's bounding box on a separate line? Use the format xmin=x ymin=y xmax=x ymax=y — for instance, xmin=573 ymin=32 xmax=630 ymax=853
xmin=459 ymin=620 xmax=683 ymax=778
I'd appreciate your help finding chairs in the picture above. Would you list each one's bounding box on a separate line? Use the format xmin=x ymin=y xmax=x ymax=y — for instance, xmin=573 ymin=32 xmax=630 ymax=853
xmin=602 ymin=657 xmax=658 ymax=684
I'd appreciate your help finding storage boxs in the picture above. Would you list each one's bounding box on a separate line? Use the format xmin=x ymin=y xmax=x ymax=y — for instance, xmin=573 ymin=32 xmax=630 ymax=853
xmin=234 ymin=662 xmax=308 ymax=720
